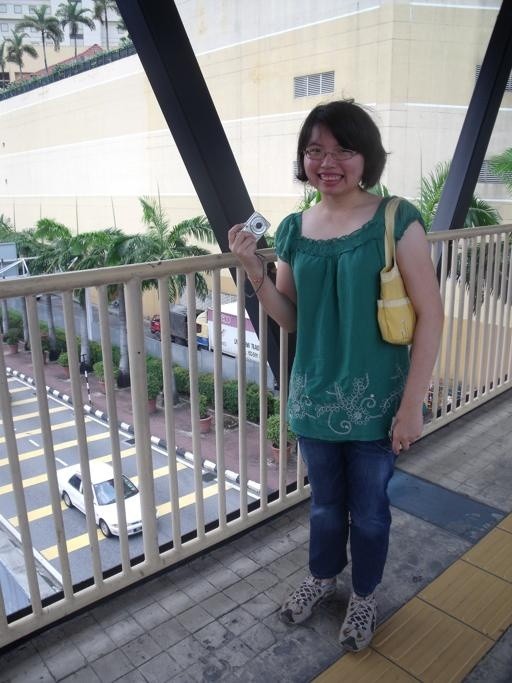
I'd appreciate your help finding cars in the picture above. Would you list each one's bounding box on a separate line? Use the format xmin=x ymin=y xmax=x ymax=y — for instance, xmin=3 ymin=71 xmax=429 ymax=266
xmin=58 ymin=457 xmax=157 ymax=537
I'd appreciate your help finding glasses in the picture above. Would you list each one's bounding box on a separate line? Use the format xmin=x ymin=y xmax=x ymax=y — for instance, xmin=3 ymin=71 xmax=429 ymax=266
xmin=303 ymin=147 xmax=358 ymax=160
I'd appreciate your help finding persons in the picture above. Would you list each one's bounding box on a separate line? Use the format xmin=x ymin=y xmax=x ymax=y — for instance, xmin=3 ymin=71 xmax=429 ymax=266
xmin=228 ymin=99 xmax=444 ymax=653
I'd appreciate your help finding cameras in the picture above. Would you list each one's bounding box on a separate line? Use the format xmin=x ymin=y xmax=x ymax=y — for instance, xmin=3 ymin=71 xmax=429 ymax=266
xmin=240 ymin=211 xmax=271 ymax=243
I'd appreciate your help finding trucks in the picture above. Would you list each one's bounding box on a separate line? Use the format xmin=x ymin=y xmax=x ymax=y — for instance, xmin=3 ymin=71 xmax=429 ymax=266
xmin=193 ymin=300 xmax=270 ymax=368
xmin=150 ymin=304 xmax=205 ymax=344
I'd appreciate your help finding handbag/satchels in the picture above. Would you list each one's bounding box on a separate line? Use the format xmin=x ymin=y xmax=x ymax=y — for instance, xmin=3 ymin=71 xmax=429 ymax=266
xmin=377 ymin=196 xmax=416 ymax=345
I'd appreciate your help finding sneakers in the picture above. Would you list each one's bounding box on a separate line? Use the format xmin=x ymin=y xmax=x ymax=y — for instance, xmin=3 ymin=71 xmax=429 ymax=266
xmin=338 ymin=590 xmax=377 ymax=652
xmin=278 ymin=576 xmax=336 ymax=625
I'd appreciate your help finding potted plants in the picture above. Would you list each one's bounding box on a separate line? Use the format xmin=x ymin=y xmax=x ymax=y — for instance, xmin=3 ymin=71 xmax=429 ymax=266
xmin=146 ymin=374 xmax=161 ymax=413
xmin=198 ymin=393 xmax=212 ymax=434
xmin=40 ymin=332 xmax=50 ymax=363
xmin=93 ymin=361 xmax=119 ymax=395
xmin=267 ymin=412 xmax=296 ymax=464
xmin=4 ymin=327 xmax=21 ymax=355
xmin=58 ymin=352 xmax=70 ymax=377
